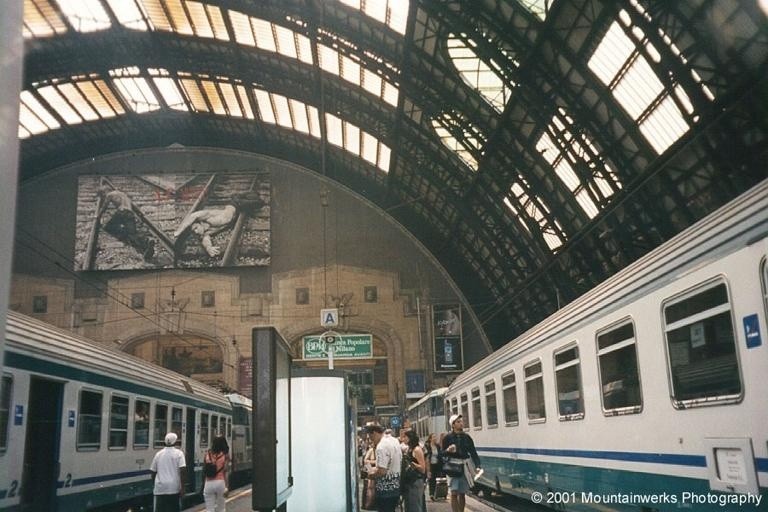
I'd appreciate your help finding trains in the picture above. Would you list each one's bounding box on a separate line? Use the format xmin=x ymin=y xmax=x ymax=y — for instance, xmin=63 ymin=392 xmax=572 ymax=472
xmin=0 ymin=308 xmax=253 ymax=512
xmin=407 ymin=176 xmax=768 ymax=512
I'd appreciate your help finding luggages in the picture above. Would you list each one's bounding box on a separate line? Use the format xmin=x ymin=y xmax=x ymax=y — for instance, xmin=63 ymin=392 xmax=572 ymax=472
xmin=435 ymin=476 xmax=448 ymax=499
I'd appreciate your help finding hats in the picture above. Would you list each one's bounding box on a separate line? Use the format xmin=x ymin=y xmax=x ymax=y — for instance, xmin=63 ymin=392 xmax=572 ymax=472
xmin=448 ymin=414 xmax=462 ymax=426
xmin=164 ymin=431 xmax=178 ymax=445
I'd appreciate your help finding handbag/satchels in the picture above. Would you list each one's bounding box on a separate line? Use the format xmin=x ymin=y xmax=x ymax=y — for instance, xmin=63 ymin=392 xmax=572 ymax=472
xmin=361 ymin=477 xmax=378 ymax=510
xmin=442 ymin=449 xmax=463 ymax=478
xmin=400 ymin=454 xmax=420 ymax=481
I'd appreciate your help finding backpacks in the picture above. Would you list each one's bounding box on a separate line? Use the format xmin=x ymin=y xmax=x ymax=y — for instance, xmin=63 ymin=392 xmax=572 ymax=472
xmin=202 ymin=461 xmax=217 ymax=477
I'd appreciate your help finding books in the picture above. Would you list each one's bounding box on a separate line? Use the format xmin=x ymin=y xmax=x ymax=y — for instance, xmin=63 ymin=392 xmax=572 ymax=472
xmin=473 ymin=468 xmax=483 ymax=482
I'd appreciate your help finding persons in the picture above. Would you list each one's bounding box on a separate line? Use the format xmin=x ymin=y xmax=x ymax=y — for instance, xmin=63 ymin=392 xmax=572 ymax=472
xmin=94 ymin=182 xmax=154 ymax=264
xmin=134 ymin=405 xmax=148 ymax=422
xmin=356 ymin=418 xmax=446 ymax=512
xmin=148 ymin=431 xmax=187 ymax=511
xmin=200 ymin=435 xmax=231 ymax=512
xmin=173 ymin=188 xmax=266 ymax=259
xmin=439 ymin=413 xmax=481 ymax=511
